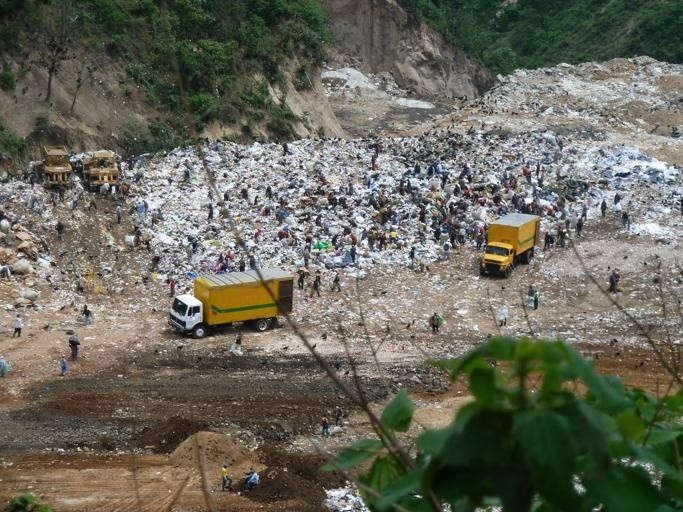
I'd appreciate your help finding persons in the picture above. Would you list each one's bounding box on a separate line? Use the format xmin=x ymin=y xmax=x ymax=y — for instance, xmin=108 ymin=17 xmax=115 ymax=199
xmin=333 ymin=405 xmax=343 ymax=427
xmin=532 ymin=289 xmax=537 ymax=310
xmin=181 ymin=138 xmax=481 ymax=298
xmin=58 ymin=354 xmax=66 ymax=376
xmin=498 ymin=305 xmax=507 ymax=327
xmin=527 ymin=284 xmax=534 ymax=308
xmin=321 ymin=414 xmax=329 ymax=437
xmin=0 ymin=355 xmax=10 ymax=377
xmin=1 ymin=161 xmax=179 ymax=297
xmin=241 ymin=467 xmax=253 ymax=483
xmin=11 ymin=314 xmax=21 ymax=337
xmin=458 ymin=138 xmax=632 ymax=294
xmin=67 ymin=332 xmax=80 ymax=359
xmin=81 ymin=305 xmax=92 ymax=325
xmin=221 ymin=464 xmax=232 ymax=490
xmin=429 ymin=310 xmax=438 ymax=331
xmin=228 ymin=331 xmax=242 ymax=356
xmin=246 ymin=470 xmax=260 ymax=489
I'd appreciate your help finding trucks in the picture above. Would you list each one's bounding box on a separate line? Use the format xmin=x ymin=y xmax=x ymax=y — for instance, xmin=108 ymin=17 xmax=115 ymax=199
xmin=479 ymin=209 xmax=543 ymax=280
xmin=161 ymin=264 xmax=293 ymax=340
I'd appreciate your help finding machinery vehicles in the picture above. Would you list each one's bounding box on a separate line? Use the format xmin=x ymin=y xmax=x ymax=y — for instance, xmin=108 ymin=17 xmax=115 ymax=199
xmin=39 ymin=143 xmax=76 ymax=189
xmin=80 ymin=149 xmax=122 ymax=193
